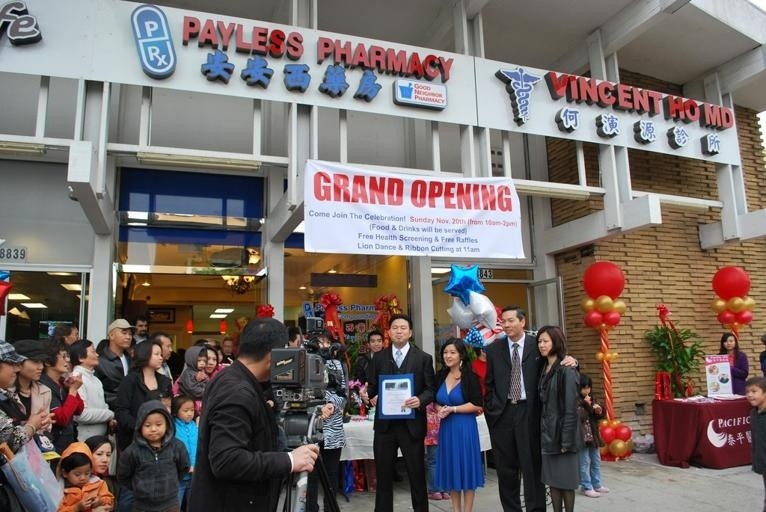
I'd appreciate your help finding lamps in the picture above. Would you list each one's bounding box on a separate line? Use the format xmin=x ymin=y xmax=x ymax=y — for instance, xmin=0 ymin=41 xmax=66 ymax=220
xmin=213 ymin=249 xmax=260 ymax=295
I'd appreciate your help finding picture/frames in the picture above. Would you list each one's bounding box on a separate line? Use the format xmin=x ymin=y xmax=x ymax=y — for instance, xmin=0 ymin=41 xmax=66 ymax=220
xmin=148 ymin=307 xmax=176 ymax=323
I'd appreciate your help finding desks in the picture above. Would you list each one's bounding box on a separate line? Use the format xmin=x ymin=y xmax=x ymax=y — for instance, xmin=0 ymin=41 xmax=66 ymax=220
xmin=338 ymin=408 xmax=492 ymax=492
xmin=652 ymin=396 xmax=754 ymax=469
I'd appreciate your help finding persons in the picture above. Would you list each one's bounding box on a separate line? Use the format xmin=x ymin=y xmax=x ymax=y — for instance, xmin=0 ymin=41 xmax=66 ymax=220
xmin=745 ymin=334 xmax=766 ymax=512
xmin=1 ymin=304 xmax=611 ymax=511
xmin=716 ymin=332 xmax=749 ymax=396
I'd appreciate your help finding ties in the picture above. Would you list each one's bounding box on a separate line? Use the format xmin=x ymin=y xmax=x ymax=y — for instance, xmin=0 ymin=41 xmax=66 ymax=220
xmin=395 ymin=351 xmax=402 ymax=368
xmin=508 ymin=343 xmax=520 ymax=402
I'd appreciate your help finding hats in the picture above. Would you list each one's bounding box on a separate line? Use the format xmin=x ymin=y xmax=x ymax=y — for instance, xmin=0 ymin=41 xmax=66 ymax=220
xmin=34 ymin=434 xmax=61 ymax=460
xmin=0 ymin=340 xmax=28 ymax=363
xmin=107 ymin=319 xmax=135 ymax=334
xmin=13 ymin=339 xmax=50 ymax=360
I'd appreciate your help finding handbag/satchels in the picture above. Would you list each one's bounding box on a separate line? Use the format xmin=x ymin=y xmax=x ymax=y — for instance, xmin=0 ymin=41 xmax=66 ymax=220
xmin=0 ymin=436 xmax=64 ymax=512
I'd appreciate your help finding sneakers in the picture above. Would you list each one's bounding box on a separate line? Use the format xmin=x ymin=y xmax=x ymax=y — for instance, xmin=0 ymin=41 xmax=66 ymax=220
xmin=584 ymin=490 xmax=600 ymax=498
xmin=431 ymin=494 xmax=441 ymax=500
xmin=441 ymin=495 xmax=449 ymax=499
xmin=595 ymin=487 xmax=609 ymax=493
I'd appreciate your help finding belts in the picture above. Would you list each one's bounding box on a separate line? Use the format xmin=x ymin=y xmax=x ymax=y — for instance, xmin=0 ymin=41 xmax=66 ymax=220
xmin=507 ymin=400 xmax=526 ymax=405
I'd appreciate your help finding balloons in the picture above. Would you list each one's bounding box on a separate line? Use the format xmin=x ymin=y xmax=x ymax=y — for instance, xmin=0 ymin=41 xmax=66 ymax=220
xmin=712 ymin=266 xmax=755 ymax=330
xmin=0 ymin=278 xmax=15 ymax=317
xmin=596 ymin=418 xmax=634 ymax=461
xmin=595 ymin=351 xmax=619 ymax=363
xmin=579 ymin=262 xmax=627 ymax=329
xmin=443 ymin=263 xmax=505 ymax=351
xmin=0 ymin=270 xmax=11 ymax=280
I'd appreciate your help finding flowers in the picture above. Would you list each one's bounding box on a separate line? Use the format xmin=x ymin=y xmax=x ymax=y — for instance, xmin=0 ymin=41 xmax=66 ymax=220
xmin=348 ymin=379 xmax=371 ymax=415
